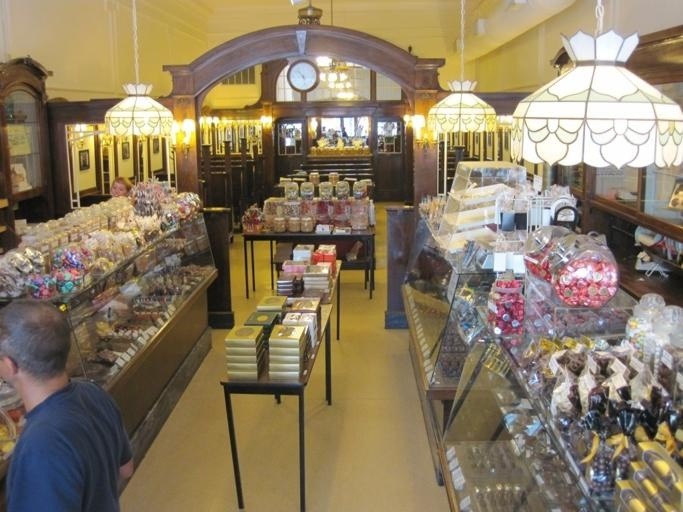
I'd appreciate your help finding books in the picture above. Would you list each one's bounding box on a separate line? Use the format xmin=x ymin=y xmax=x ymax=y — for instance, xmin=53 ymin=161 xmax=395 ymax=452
xmin=219 ymin=241 xmax=340 ymax=390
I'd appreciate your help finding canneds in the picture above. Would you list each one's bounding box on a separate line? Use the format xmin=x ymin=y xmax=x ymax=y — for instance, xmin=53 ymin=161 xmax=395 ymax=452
xmin=285 ymin=172 xmax=372 ymax=202
xmin=274 ymin=216 xmax=313 ymax=233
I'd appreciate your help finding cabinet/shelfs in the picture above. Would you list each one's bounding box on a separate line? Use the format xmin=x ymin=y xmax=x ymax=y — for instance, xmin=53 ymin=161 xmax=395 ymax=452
xmin=0 ymin=57 xmax=55 ymax=258
xmin=550 ymin=25 xmax=683 ymax=307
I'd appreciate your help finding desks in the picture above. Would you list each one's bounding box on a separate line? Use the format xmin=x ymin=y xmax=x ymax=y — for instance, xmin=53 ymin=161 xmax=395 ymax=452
xmin=276 ymin=242 xmax=343 ymax=342
xmin=218 ymin=301 xmax=336 ymax=512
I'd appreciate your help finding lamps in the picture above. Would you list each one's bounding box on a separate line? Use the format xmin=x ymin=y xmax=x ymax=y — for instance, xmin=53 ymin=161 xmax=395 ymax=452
xmin=425 ymin=1 xmax=500 ymax=139
xmin=507 ymin=3 xmax=683 ymax=171
xmin=103 ymin=0 xmax=174 ymax=139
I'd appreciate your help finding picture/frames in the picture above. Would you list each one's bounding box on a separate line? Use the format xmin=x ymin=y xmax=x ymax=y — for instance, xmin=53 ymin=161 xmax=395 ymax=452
xmin=78 ymin=150 xmax=89 ymax=171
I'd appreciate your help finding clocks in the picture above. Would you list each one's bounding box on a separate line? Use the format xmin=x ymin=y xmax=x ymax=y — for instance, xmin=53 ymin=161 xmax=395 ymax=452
xmin=286 ymin=59 xmax=320 ymax=93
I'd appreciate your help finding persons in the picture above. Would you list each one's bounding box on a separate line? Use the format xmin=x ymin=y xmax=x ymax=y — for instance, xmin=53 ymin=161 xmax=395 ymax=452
xmin=0 ymin=296 xmax=134 ymax=510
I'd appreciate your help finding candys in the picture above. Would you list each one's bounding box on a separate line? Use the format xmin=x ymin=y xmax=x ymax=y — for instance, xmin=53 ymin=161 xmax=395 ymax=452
xmin=23 ymin=246 xmax=88 ymax=300
xmin=491 ymin=240 xmax=682 ymax=512
xmin=129 ymin=179 xmax=201 ymax=226
